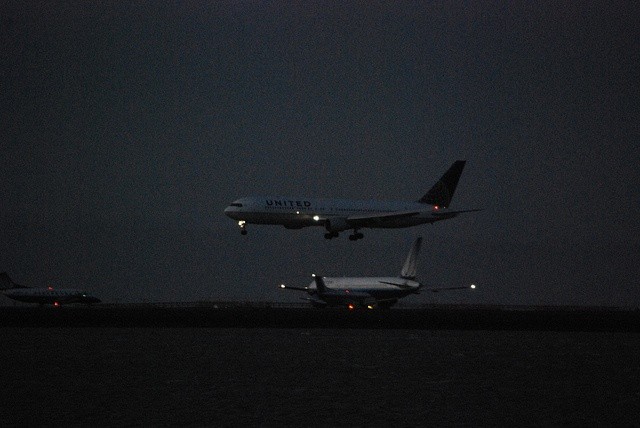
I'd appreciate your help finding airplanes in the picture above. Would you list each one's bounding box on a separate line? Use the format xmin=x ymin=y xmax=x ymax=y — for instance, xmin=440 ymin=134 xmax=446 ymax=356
xmin=224 ymin=161 xmax=481 ymax=241
xmin=278 ymin=237 xmax=476 ymax=309
xmin=2 ymin=271 xmax=101 ymax=307
